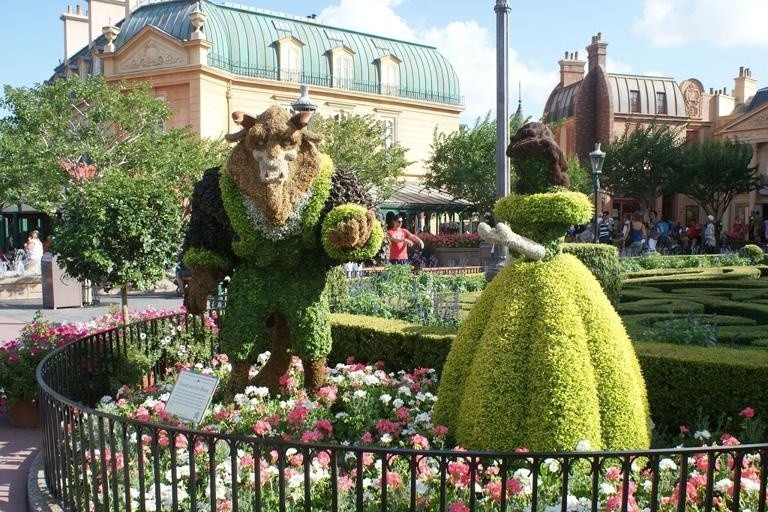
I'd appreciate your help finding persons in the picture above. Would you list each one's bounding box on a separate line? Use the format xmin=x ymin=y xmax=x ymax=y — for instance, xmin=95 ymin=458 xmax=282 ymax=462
xmin=23 ymin=230 xmax=44 ymax=277
xmin=566 ymin=209 xmax=768 ymax=257
xmin=386 ymin=215 xmax=425 ymax=264
xmin=174 ymin=245 xmax=193 ymax=298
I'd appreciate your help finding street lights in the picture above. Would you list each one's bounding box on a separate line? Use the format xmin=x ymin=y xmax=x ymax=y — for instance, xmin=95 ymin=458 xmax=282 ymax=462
xmin=586 ymin=140 xmax=606 ymax=247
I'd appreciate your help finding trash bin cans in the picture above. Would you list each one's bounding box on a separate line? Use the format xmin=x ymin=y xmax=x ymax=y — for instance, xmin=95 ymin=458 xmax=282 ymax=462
xmin=41 ymin=251 xmax=84 ymax=310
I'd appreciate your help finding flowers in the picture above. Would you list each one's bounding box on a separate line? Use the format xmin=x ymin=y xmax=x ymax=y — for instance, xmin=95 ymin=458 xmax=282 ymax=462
xmin=4 ymin=306 xmax=194 ymax=400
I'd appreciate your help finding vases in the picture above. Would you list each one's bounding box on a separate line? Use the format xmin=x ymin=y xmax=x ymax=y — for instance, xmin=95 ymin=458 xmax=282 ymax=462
xmin=6 ymin=392 xmax=46 ymax=427
